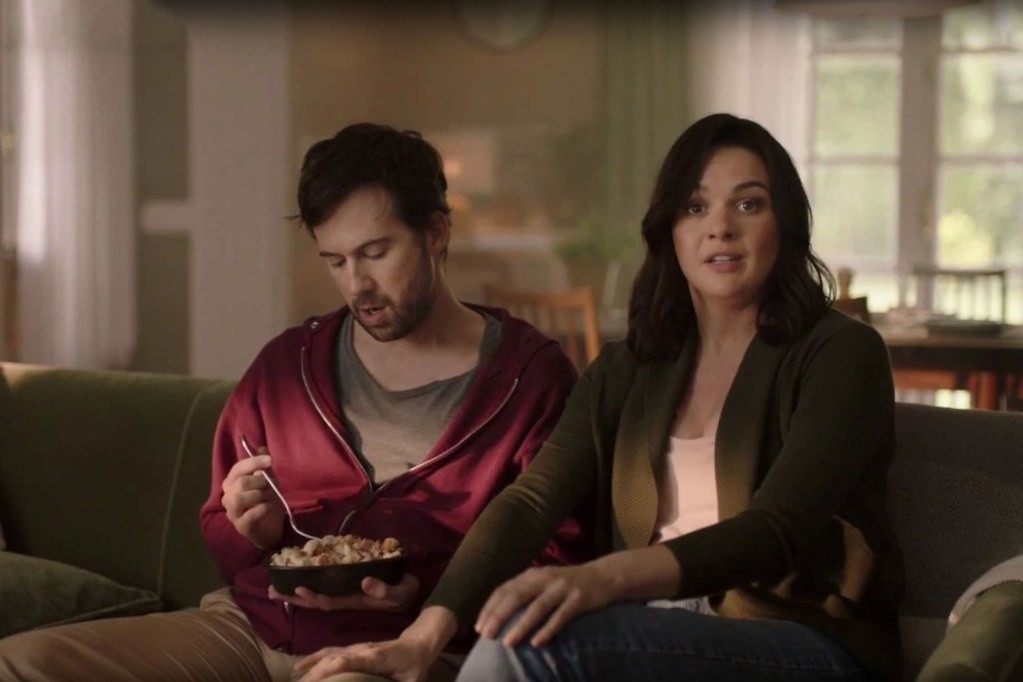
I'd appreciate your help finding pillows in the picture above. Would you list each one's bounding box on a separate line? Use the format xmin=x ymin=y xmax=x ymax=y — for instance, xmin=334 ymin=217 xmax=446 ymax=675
xmin=0 ymin=550 xmax=162 ymax=640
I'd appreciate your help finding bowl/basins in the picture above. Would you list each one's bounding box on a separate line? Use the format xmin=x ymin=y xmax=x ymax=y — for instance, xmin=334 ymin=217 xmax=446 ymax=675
xmin=262 ymin=546 xmax=409 ymax=598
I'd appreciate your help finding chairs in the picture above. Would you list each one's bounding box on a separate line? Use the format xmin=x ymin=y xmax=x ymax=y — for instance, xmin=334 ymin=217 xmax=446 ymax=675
xmin=912 ymin=266 xmax=1009 ymax=323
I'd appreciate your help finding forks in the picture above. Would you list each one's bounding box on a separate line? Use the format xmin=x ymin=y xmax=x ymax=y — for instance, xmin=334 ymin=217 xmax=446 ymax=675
xmin=239 ymin=432 xmax=322 ymax=542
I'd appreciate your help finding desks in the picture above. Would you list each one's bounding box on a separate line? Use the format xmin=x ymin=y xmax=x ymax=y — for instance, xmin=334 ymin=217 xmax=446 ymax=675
xmin=859 ymin=311 xmax=1023 ymax=414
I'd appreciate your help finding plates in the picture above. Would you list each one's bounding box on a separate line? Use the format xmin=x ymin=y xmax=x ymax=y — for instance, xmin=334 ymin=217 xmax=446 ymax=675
xmin=920 ymin=318 xmax=1009 ymax=338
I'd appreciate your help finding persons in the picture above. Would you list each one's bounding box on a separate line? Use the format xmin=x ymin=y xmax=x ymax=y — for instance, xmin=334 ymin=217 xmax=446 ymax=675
xmin=297 ymin=112 xmax=899 ymax=682
xmin=0 ymin=120 xmax=608 ymax=682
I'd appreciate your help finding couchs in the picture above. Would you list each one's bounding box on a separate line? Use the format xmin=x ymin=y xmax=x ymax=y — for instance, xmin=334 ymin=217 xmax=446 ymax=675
xmin=0 ymin=360 xmax=1023 ymax=682
